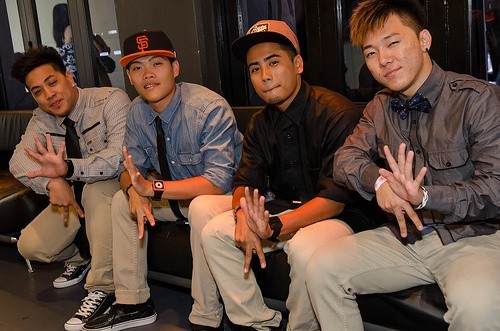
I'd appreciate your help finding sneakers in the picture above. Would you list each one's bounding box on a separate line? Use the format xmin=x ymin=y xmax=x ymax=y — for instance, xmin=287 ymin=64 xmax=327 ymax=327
xmin=64 ymin=289 xmax=116 ymax=331
xmin=53 ymin=262 xmax=91 ymax=288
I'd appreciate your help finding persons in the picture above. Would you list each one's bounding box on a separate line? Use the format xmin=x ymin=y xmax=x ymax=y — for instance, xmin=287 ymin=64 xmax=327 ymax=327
xmin=83 ymin=30 xmax=244 ymax=331
xmin=305 ymin=0 xmax=500 ymax=331
xmin=200 ymin=20 xmax=389 ymax=331
xmin=9 ymin=46 xmax=132 ymax=331
xmin=53 ymin=4 xmax=78 ymax=86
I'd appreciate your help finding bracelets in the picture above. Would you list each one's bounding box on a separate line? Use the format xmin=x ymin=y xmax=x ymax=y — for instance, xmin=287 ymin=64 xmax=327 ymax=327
xmin=233 ymin=206 xmax=241 ymax=224
xmin=125 ymin=183 xmax=133 ymax=198
xmin=411 ymin=186 xmax=428 ymax=210
xmin=374 ymin=172 xmax=393 ymax=191
xmin=64 ymin=159 xmax=74 ymax=179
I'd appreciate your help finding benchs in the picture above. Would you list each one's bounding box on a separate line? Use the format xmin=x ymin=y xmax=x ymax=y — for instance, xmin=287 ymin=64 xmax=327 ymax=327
xmin=0 ymin=102 xmax=451 ymax=331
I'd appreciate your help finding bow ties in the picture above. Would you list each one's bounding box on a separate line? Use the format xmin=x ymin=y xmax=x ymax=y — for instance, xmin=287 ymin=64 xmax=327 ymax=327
xmin=390 ymin=94 xmax=432 ymax=119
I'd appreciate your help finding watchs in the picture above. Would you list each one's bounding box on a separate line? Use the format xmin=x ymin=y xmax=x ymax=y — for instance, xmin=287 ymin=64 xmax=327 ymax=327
xmin=150 ymin=180 xmax=164 ymax=202
xmin=267 ymin=217 xmax=282 ymax=243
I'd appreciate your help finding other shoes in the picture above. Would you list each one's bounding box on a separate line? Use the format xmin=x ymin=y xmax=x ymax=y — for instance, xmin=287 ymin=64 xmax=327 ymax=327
xmin=82 ymin=297 xmax=158 ymax=331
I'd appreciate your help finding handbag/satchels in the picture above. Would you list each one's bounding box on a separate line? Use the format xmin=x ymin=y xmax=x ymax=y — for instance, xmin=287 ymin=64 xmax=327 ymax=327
xmin=94 ymin=44 xmax=116 ymax=73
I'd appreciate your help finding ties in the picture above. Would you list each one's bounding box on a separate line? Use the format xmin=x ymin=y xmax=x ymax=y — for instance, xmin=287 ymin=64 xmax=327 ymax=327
xmin=62 ymin=117 xmax=91 ymax=261
xmin=154 ymin=115 xmax=188 ymax=221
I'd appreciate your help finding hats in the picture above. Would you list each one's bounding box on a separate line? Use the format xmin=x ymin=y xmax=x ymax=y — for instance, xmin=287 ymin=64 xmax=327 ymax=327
xmin=118 ymin=29 xmax=177 ymax=67
xmin=230 ymin=18 xmax=301 ymax=58
